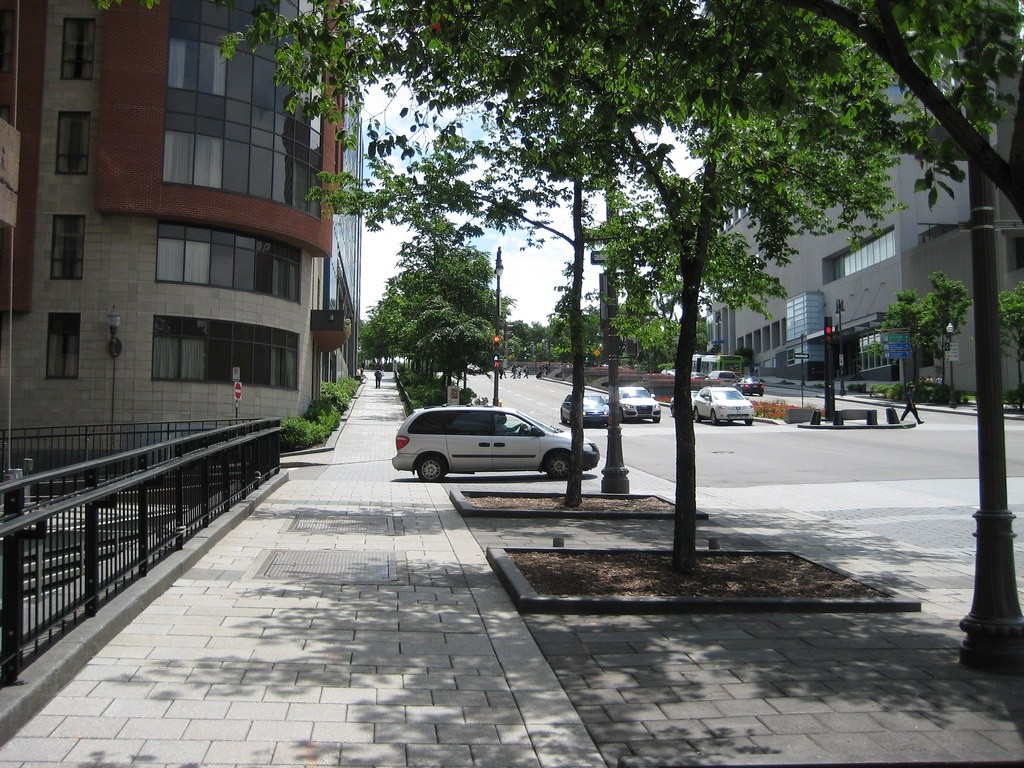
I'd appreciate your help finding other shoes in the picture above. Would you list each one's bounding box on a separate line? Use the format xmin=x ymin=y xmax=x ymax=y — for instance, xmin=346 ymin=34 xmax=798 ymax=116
xmin=918 ymin=421 xmax=924 ymax=424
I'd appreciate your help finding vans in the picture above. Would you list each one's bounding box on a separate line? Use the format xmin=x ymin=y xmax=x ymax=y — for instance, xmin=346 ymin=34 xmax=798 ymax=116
xmin=705 ymin=370 xmax=736 ymax=386
xmin=392 ymin=403 xmax=600 ymax=482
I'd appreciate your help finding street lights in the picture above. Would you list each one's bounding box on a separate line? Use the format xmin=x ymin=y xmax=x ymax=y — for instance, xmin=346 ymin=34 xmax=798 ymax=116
xmin=492 ymin=246 xmax=504 ymax=408
xmin=946 ymin=322 xmax=957 ymax=408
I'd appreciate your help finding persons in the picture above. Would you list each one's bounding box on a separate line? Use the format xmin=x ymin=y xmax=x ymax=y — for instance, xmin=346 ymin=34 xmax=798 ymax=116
xmin=536 ymin=362 xmax=549 ymax=379
xmin=374 ymin=367 xmax=382 ymax=389
xmin=899 ymin=385 xmax=924 ymax=424
xmin=567 ymin=361 xmax=573 ymax=368
xmin=499 ymin=364 xmax=530 ymax=379
xmin=361 ymin=362 xmax=364 ymax=369
xmin=754 ymin=364 xmax=759 ymax=377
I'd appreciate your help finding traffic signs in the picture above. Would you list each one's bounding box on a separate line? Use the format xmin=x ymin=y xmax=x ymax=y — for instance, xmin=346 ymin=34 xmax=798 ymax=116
xmin=881 ymin=334 xmax=911 ymax=359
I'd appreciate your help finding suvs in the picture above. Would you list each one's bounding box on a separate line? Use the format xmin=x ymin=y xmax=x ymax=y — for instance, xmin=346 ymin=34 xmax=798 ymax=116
xmin=693 ymin=386 xmax=754 ymax=426
xmin=605 ymin=387 xmax=660 ymax=423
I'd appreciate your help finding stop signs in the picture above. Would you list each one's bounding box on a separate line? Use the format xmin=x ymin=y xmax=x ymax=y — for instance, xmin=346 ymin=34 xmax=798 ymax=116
xmin=234 ymin=382 xmax=242 ymax=400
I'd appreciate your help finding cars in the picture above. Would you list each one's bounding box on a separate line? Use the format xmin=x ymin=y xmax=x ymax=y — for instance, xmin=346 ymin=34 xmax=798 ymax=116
xmin=561 ymin=393 xmax=610 ymax=428
xmin=670 ymin=391 xmax=702 ymax=418
xmin=661 ymin=368 xmax=675 ymax=377
xmin=735 ymin=377 xmax=764 ymax=396
xmin=691 ymin=372 xmax=711 ymax=380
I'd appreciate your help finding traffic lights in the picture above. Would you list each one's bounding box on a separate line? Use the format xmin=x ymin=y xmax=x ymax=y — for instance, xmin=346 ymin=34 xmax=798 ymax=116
xmin=493 ymin=335 xmax=500 ymax=369
xmin=824 ymin=317 xmax=833 ymax=351
